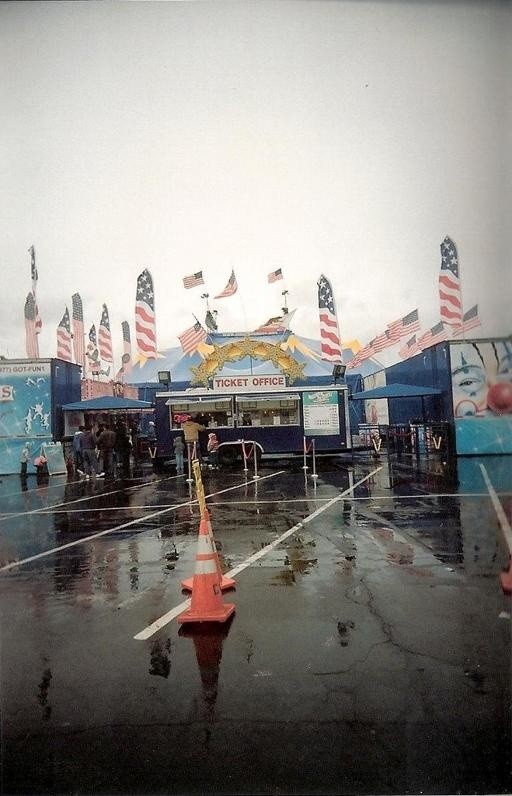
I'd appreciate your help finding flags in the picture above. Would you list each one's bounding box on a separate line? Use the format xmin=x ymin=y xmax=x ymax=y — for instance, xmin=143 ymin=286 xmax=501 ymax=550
xmin=23 ymin=242 xmax=156 ymax=378
xmin=315 ymin=274 xmax=342 ymax=369
xmin=268 ymin=268 xmax=284 ymax=284
xmin=344 ymin=308 xmax=481 ymax=369
xmin=177 ymin=324 xmax=207 ymax=352
xmin=213 ymin=272 xmax=240 ymax=299
xmin=439 ymin=234 xmax=464 ymax=326
xmin=180 ymin=272 xmax=205 ymax=289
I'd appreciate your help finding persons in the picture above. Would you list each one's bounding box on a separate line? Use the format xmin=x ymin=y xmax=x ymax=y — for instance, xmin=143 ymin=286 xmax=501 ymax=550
xmin=204 ymin=432 xmax=221 ymax=470
xmin=450 ymin=340 xmax=511 ymax=418
xmin=180 ymin=417 xmax=205 ymax=460
xmin=172 ymin=436 xmax=185 ymax=471
xmin=19 ymin=441 xmax=30 ymax=475
xmin=71 ymin=417 xmax=157 ymax=478
xmin=35 ymin=456 xmax=48 ymax=474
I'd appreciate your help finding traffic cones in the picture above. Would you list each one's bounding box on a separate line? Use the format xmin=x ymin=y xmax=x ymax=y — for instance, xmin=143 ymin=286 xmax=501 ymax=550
xmin=500 ymin=554 xmax=512 ymax=593
xmin=178 ymin=503 xmax=236 ymax=627
xmin=175 ymin=613 xmax=244 ymax=746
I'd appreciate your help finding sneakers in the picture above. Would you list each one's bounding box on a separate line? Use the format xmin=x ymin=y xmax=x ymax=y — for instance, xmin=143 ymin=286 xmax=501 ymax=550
xmin=176 ymin=466 xmax=219 ymax=472
xmin=20 ymin=462 xmax=124 ymax=480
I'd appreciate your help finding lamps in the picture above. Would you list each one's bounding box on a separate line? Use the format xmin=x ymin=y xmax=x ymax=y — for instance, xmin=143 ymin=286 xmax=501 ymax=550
xmin=333 ymin=364 xmax=347 ymax=385
xmin=156 ymin=369 xmax=171 ymax=389
